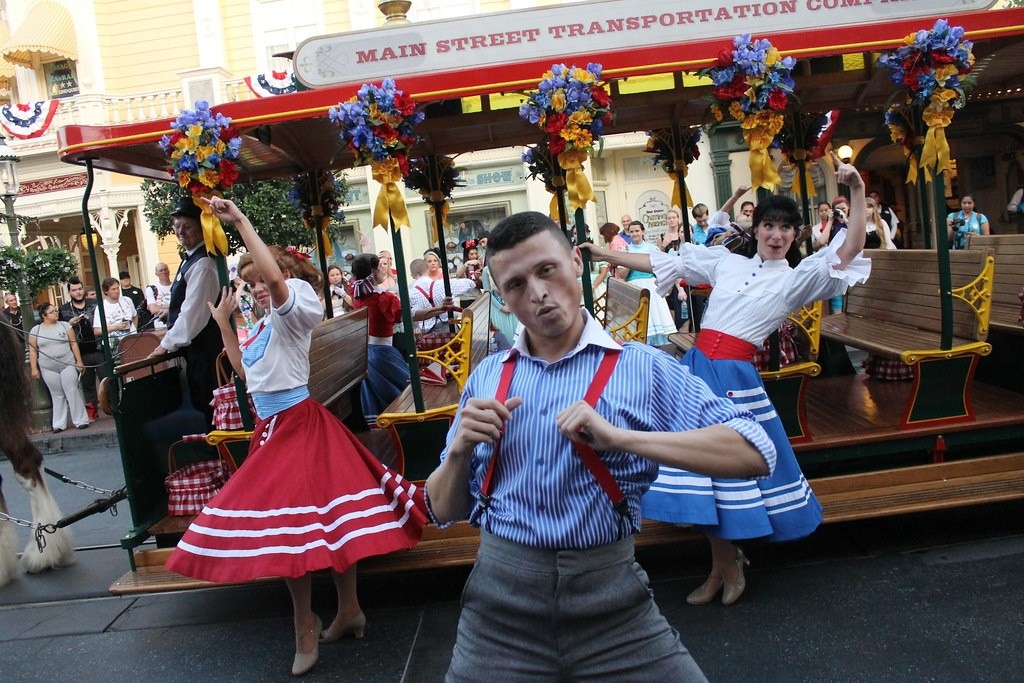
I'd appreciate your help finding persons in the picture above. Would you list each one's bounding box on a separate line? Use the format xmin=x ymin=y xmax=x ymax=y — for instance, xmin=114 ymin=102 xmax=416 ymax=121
xmin=1007 ymin=188 xmax=1024 ymax=234
xmin=424 ymin=212 xmax=777 ymax=682
xmin=947 ymin=195 xmax=990 ymax=249
xmin=163 ymin=195 xmax=430 ymax=674
xmin=0 ymin=189 xmax=900 ymax=434
xmin=575 ymin=151 xmax=872 ymax=605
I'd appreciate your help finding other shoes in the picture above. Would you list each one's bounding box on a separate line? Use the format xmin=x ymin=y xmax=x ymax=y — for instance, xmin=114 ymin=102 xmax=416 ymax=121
xmin=79 ymin=424 xmax=88 ymax=429
xmin=53 ymin=428 xmax=62 ymax=433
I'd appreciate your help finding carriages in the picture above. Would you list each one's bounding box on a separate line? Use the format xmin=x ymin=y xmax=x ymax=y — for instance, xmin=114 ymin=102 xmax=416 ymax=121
xmin=0 ymin=1 xmax=1024 ymax=597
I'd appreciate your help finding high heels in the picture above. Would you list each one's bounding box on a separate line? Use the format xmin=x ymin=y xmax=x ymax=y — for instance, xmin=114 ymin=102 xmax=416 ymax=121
xmin=291 ymin=614 xmax=325 ymax=676
xmin=686 ymin=573 xmax=723 ymax=606
xmin=721 ymin=543 xmax=751 ymax=606
xmin=319 ymin=611 xmax=365 ymax=638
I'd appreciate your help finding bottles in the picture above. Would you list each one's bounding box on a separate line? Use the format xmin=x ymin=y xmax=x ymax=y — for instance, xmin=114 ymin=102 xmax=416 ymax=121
xmin=681 ymin=300 xmax=688 ymax=319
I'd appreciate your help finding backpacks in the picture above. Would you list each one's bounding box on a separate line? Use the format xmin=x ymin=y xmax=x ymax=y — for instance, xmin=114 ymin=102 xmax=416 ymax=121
xmin=136 ymin=285 xmax=158 ymax=329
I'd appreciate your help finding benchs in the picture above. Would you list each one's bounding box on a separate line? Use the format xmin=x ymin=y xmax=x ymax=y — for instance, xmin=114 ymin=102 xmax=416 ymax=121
xmin=207 ymin=234 xmax=1024 ymax=491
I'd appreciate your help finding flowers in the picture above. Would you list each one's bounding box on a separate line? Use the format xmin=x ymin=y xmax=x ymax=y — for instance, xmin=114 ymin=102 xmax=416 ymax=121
xmin=875 ymin=17 xmax=976 ymax=177
xmin=288 ymin=170 xmax=346 ymax=262
xmin=519 ymin=62 xmax=617 ymax=215
xmin=401 ymin=153 xmax=469 ymax=244
xmin=772 ymin=112 xmax=829 ymax=199
xmin=159 ymin=100 xmax=244 ymax=258
xmin=328 ymin=76 xmax=425 ymax=233
xmin=521 ymin=143 xmax=571 ymax=225
xmin=884 ymin=104 xmax=933 ymax=186
xmin=693 ymin=31 xmax=803 ymax=193
xmin=642 ymin=125 xmax=702 ymax=210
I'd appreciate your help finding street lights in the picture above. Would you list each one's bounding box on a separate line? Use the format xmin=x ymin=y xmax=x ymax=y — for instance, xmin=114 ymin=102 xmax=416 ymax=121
xmin=0 ymin=155 xmax=54 ymax=431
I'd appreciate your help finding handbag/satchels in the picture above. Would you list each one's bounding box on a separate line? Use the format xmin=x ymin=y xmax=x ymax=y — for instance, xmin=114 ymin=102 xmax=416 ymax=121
xmin=74 ymin=318 xmax=98 ymax=353
xmin=417 ymin=307 xmax=464 ymax=367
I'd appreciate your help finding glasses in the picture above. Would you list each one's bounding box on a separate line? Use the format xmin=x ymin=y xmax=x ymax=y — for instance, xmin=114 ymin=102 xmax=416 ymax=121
xmin=45 ymin=308 xmax=58 ymax=314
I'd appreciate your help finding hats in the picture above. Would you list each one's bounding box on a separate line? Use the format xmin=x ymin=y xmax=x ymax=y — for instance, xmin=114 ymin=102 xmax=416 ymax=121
xmin=171 ymin=197 xmax=202 ymax=220
xmin=944 ymin=185 xmax=959 ymax=200
xmin=478 ymin=231 xmax=489 ymax=241
xmin=891 ymin=206 xmax=902 ymax=212
xmin=120 ymin=272 xmax=130 ymax=280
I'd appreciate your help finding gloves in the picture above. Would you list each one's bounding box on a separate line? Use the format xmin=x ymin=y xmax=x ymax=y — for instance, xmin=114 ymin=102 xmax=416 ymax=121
xmin=330 ymin=284 xmax=335 ymax=296
xmin=464 ymin=260 xmax=480 ymax=267
xmin=335 ymin=285 xmax=347 ymax=298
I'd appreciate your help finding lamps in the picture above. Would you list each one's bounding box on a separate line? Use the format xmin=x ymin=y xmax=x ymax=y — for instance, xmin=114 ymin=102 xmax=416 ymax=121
xmin=838 ymin=145 xmax=853 ymax=164
xmin=80 ymin=227 xmax=98 ymax=250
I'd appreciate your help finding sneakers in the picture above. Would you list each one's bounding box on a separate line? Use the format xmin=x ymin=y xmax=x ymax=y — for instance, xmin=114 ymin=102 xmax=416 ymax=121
xmin=85 ymin=400 xmax=99 ymax=422
